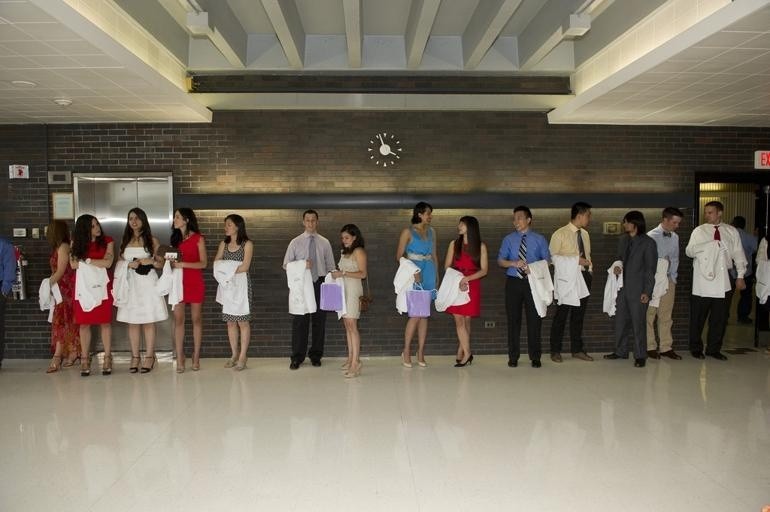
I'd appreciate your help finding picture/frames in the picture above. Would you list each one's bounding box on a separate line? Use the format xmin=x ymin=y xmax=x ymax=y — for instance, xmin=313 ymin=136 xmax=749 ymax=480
xmin=52 ymin=192 xmax=75 ymax=222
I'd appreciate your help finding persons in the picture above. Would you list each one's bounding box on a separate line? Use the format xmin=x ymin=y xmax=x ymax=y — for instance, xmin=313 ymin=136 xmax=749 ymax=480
xmin=497 ymin=206 xmax=552 ymax=368
xmin=445 ymin=216 xmax=488 ymax=368
xmin=603 ymin=210 xmax=659 ymax=367
xmin=213 ymin=214 xmax=256 ymax=372
xmin=166 ymin=207 xmax=208 ymax=373
xmin=42 ymin=219 xmax=82 ymax=371
xmin=650 ymin=206 xmax=685 ymax=360
xmin=112 ymin=208 xmax=169 ymax=374
xmin=396 ymin=201 xmax=440 ymax=370
xmin=728 ymin=214 xmax=758 ymax=324
xmin=554 ymin=204 xmax=595 ymax=364
xmin=282 ymin=210 xmax=336 ymax=369
xmin=68 ymin=214 xmax=115 ymax=375
xmin=333 ymin=224 xmax=369 ymax=379
xmin=0 ymin=235 xmax=17 ymax=367
xmin=686 ymin=200 xmax=747 ymax=360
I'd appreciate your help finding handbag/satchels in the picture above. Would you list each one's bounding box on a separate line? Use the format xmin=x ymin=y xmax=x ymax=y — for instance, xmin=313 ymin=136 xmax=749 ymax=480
xmin=406 ymin=280 xmax=432 ymax=318
xmin=359 ymin=276 xmax=373 ymax=311
xmin=319 ymin=279 xmax=343 ymax=311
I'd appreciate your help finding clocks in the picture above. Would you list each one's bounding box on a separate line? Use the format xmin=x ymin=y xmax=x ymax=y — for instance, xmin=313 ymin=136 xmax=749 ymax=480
xmin=366 ymin=127 xmax=404 ymax=169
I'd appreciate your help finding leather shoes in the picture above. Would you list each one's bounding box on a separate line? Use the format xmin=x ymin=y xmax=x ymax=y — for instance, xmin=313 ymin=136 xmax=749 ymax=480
xmin=401 ymin=352 xmax=412 ymax=368
xmin=508 ymin=351 xmax=594 ymax=368
xmin=604 ymin=349 xmax=727 ymax=367
xmin=736 ymin=316 xmax=753 ymax=324
xmin=191 ymin=351 xmax=200 ymax=371
xmin=415 ymin=351 xmax=426 ymax=367
xmin=176 ymin=354 xmax=186 ymax=373
xmin=290 ymin=360 xmax=302 ymax=369
xmin=311 ymin=360 xmax=321 ymax=367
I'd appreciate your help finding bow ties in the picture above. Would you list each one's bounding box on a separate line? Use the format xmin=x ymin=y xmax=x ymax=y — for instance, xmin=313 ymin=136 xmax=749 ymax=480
xmin=663 ymin=230 xmax=671 ymax=237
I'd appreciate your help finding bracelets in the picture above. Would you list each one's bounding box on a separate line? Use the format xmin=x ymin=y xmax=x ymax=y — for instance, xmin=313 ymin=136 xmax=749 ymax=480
xmin=343 ymin=270 xmax=348 ymax=275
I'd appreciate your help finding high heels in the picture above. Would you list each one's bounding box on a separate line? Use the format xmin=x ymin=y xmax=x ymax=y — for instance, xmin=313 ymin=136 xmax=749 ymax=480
xmin=140 ymin=356 xmax=155 ymax=373
xmin=129 ymin=356 xmax=141 ymax=373
xmin=456 ymin=350 xmax=464 ymax=363
xmin=46 ymin=350 xmax=113 ymax=376
xmin=341 ymin=360 xmax=352 ymax=370
xmin=343 ymin=360 xmax=363 ymax=378
xmin=454 ymin=353 xmax=473 ymax=367
xmin=224 ymin=356 xmax=239 ymax=368
xmin=233 ymin=356 xmax=248 ymax=371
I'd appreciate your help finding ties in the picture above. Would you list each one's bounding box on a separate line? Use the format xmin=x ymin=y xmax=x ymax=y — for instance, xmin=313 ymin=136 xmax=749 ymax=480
xmin=516 ymin=233 xmax=527 ymax=280
xmin=576 ymin=230 xmax=589 ymax=272
xmin=308 ymin=234 xmax=319 ymax=283
xmin=713 ymin=226 xmax=720 ymax=241
